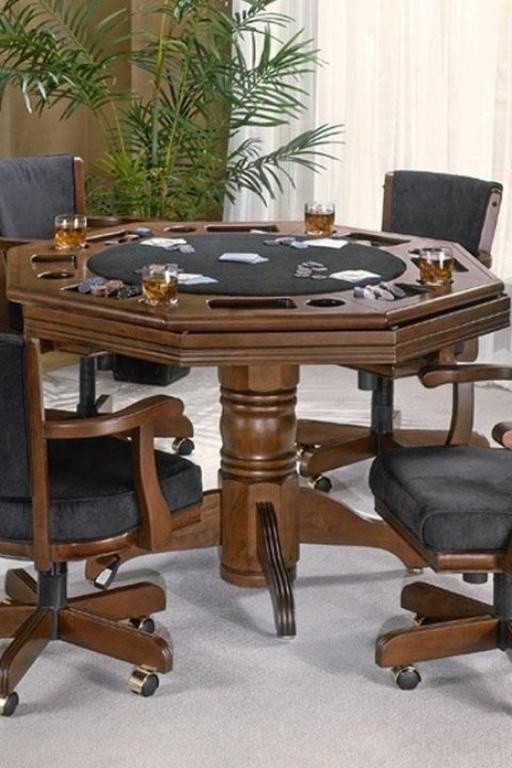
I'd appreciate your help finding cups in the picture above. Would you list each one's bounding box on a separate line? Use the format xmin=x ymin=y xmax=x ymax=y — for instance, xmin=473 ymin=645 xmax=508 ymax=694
xmin=53 ymin=212 xmax=89 ymax=251
xmin=140 ymin=263 xmax=179 ymax=307
xmin=419 ymin=244 xmax=454 ymax=286
xmin=303 ymin=200 xmax=336 ymax=237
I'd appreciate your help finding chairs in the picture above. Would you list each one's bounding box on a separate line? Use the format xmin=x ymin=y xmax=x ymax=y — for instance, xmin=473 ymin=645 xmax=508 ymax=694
xmin=0 ymin=155 xmax=511 ymax=718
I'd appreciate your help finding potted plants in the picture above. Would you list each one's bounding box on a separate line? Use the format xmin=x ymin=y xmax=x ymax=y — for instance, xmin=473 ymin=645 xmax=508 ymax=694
xmin=0 ymin=0 xmax=353 ymax=386
